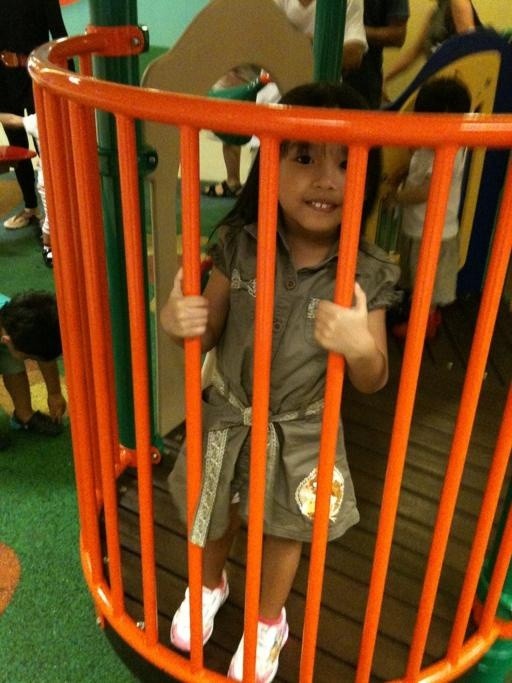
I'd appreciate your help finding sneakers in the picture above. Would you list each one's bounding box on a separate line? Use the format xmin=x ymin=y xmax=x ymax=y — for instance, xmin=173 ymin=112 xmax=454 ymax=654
xmin=8 ymin=407 xmax=65 ymax=437
xmin=172 ymin=567 xmax=230 ymax=651
xmin=2 ymin=205 xmax=42 ymax=230
xmin=226 ymin=604 xmax=290 ymax=683
xmin=393 ymin=315 xmax=442 ymax=339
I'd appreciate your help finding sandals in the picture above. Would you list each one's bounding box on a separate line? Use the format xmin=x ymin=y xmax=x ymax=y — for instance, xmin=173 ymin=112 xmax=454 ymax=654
xmin=202 ymin=182 xmax=244 ymax=197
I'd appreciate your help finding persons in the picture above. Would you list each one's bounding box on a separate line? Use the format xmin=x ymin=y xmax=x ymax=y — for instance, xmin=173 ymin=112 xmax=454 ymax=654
xmin=2 ymin=0 xmax=511 ymax=437
xmin=157 ymin=78 xmax=401 ymax=683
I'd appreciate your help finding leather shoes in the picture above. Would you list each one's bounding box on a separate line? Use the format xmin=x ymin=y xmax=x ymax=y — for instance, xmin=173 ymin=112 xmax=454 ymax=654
xmin=40 ymin=247 xmax=54 ymax=269
